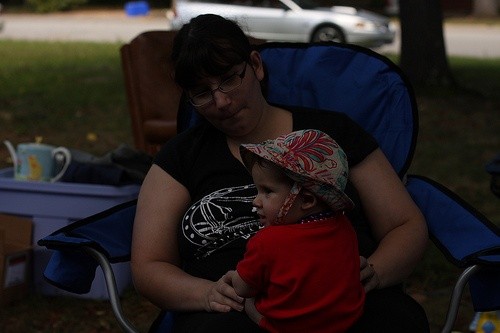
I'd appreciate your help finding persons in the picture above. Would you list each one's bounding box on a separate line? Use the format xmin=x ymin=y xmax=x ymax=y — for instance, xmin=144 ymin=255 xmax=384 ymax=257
xmin=130 ymin=14 xmax=428 ymax=333
xmin=233 ymin=129 xmax=367 ymax=333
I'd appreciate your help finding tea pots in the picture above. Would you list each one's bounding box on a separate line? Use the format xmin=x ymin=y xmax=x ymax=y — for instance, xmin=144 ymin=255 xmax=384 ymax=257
xmin=4 ymin=136 xmax=72 ymax=182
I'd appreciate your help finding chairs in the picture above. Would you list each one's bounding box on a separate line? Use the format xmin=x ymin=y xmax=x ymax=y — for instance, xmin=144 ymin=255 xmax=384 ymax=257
xmin=37 ymin=41 xmax=500 ymax=333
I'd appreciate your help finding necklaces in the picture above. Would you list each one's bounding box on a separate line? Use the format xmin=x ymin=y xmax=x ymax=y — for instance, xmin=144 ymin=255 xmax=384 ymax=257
xmin=296 ymin=209 xmax=334 ymax=222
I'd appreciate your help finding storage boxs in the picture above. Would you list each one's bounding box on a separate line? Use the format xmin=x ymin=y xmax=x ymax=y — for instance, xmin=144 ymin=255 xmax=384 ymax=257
xmin=0 ymin=167 xmax=141 ymax=300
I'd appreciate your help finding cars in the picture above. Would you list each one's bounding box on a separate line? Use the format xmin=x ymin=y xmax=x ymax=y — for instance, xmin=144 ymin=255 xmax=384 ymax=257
xmin=167 ymin=0 xmax=395 ymax=48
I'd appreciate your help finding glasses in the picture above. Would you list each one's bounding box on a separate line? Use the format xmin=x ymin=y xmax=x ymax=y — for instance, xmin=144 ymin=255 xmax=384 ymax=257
xmin=185 ymin=58 xmax=251 ymax=107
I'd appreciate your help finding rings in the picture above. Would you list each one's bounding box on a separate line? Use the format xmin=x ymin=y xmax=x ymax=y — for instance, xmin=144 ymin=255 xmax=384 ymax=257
xmin=369 ymin=263 xmax=374 ymax=274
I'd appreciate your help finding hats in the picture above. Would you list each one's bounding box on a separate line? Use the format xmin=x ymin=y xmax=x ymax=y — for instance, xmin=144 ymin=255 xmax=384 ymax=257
xmin=239 ymin=129 xmax=355 ymax=214
